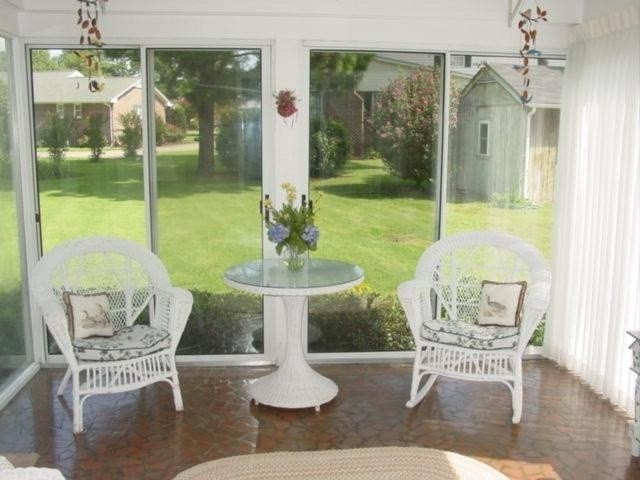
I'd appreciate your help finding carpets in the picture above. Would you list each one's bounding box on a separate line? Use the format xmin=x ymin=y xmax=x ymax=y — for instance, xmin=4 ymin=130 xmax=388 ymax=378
xmin=172 ymin=447 xmax=510 ymax=479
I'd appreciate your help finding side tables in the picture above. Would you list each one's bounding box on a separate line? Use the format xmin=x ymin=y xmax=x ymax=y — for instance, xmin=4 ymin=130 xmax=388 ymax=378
xmin=222 ymin=254 xmax=368 ymax=414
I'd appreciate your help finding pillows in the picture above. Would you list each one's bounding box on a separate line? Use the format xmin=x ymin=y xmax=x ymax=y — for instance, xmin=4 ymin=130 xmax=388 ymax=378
xmin=62 ymin=292 xmax=116 ymax=343
xmin=476 ymin=280 xmax=528 ymax=328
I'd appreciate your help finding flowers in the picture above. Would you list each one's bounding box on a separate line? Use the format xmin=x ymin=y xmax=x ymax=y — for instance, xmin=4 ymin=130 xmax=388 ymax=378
xmin=264 ymin=183 xmax=323 ymax=271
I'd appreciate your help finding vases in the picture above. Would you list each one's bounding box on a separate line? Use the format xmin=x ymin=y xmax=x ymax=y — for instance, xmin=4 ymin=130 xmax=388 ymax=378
xmin=284 ymin=240 xmax=308 ymax=273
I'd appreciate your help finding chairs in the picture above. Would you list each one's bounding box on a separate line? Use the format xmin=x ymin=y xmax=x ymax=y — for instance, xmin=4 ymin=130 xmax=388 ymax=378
xmin=394 ymin=227 xmax=555 ymax=424
xmin=26 ymin=231 xmax=195 ymax=435
